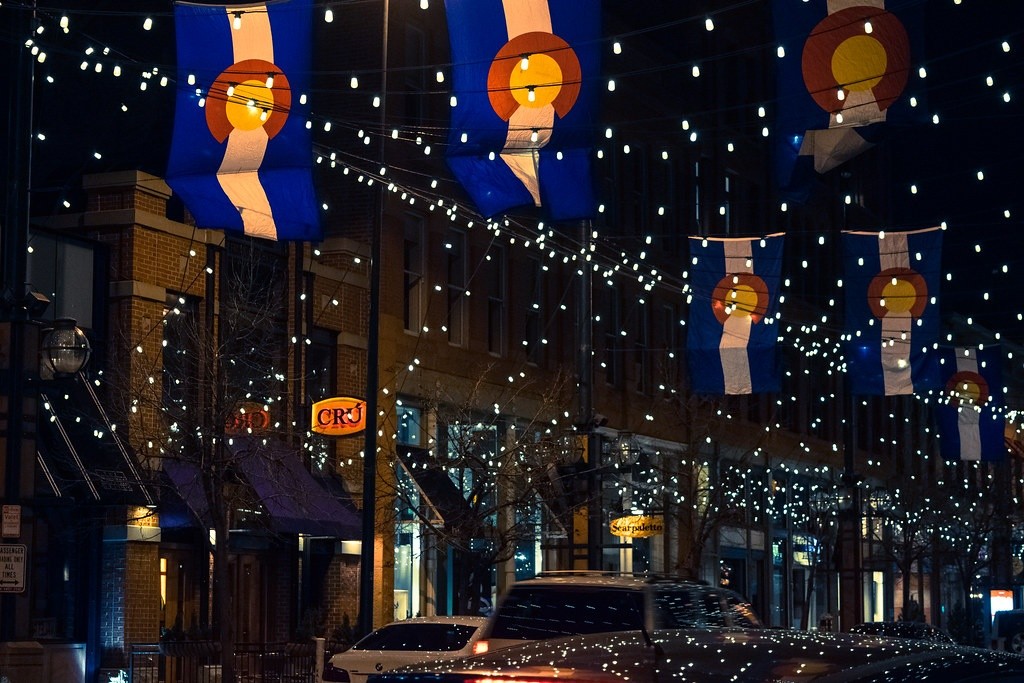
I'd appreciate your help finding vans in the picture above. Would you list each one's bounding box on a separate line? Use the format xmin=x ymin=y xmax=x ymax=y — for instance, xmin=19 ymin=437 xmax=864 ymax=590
xmin=471 ymin=569 xmax=763 ymax=656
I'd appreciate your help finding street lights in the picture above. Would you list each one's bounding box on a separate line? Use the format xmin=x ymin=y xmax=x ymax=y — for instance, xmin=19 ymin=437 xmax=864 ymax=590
xmin=805 ymin=486 xmax=895 ymax=633
xmin=567 ymin=428 xmax=642 ymax=576
xmin=1 ymin=281 xmax=95 ymax=643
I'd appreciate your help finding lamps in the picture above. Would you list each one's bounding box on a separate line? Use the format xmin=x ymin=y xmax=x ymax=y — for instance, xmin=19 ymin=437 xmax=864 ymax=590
xmin=719 ymin=571 xmax=731 ymax=588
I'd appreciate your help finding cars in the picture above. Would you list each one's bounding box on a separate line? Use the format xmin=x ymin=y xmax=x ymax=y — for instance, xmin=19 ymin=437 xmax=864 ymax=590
xmin=846 ymin=620 xmax=957 ymax=645
xmin=992 ymin=607 xmax=1024 ymax=655
xmin=321 ymin=615 xmax=492 ymax=683
xmin=365 ymin=611 xmax=920 ymax=683
